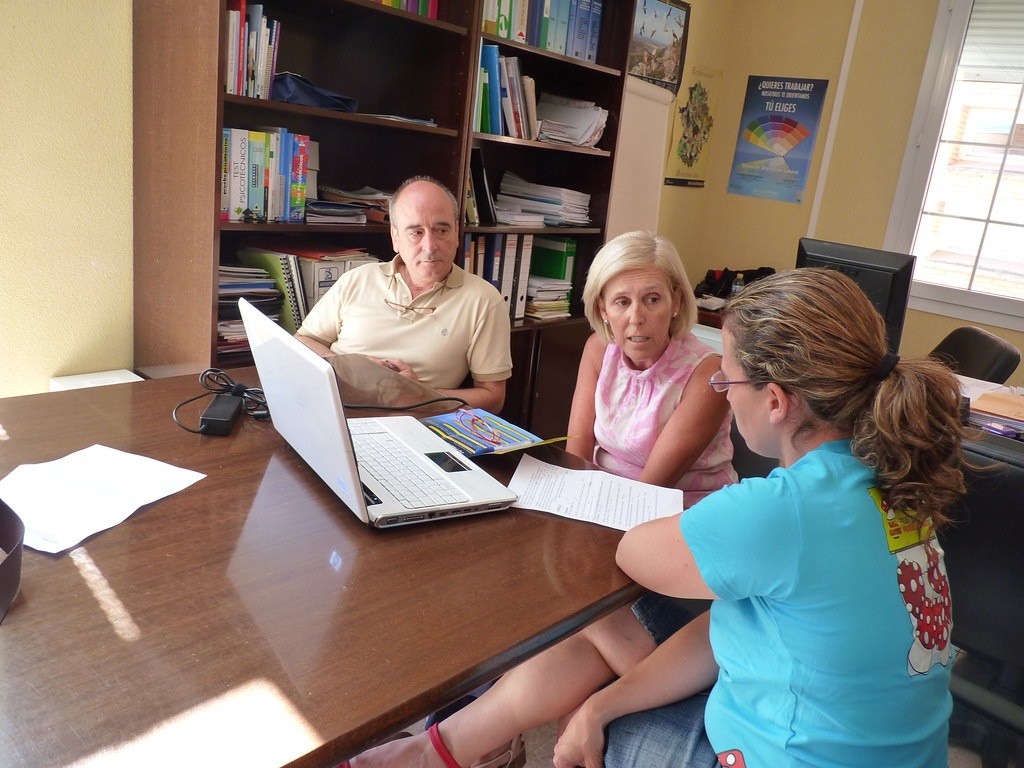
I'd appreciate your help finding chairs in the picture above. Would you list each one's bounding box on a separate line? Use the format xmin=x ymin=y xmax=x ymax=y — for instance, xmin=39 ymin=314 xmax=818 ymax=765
xmin=922 ymin=326 xmax=1021 ymax=384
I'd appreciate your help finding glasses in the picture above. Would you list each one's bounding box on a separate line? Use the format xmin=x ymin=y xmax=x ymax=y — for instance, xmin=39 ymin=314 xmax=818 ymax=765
xmin=709 ymin=367 xmax=794 ymax=397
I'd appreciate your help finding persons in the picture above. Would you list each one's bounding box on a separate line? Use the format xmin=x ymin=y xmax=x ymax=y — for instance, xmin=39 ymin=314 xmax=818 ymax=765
xmin=332 ymin=229 xmax=1008 ymax=768
xmin=292 ymin=174 xmax=512 ymax=415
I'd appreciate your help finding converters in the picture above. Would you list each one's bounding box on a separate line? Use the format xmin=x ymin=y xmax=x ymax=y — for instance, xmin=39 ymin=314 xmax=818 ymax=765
xmin=200 ymin=394 xmax=243 ymax=435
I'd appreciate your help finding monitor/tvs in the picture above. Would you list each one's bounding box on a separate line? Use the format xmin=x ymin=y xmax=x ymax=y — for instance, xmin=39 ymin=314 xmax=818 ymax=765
xmin=795 ymin=235 xmax=916 ymax=354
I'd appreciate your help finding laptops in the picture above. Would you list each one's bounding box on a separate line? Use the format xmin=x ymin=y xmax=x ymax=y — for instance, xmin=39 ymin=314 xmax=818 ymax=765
xmin=238 ymin=298 xmax=518 ymax=529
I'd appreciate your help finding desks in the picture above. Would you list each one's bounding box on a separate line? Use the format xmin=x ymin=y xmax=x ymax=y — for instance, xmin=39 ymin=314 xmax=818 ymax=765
xmin=0 ymin=365 xmax=690 ymax=768
xmin=917 ymin=376 xmax=1023 ymax=768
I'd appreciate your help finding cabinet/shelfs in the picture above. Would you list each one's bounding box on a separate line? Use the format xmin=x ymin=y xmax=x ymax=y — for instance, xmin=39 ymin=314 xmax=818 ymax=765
xmin=131 ymin=1 xmax=635 ymax=444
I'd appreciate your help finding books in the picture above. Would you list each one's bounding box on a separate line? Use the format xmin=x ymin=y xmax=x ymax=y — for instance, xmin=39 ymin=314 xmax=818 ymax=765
xmin=462 ymin=0 xmax=609 ymax=328
xmin=208 ymin=0 xmax=436 ymax=351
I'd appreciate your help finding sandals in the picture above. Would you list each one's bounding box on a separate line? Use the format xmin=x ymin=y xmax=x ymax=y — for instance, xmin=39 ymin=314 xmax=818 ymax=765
xmin=471 ymin=730 xmax=529 ymax=768
xmin=339 ymin=721 xmax=462 ymax=768
